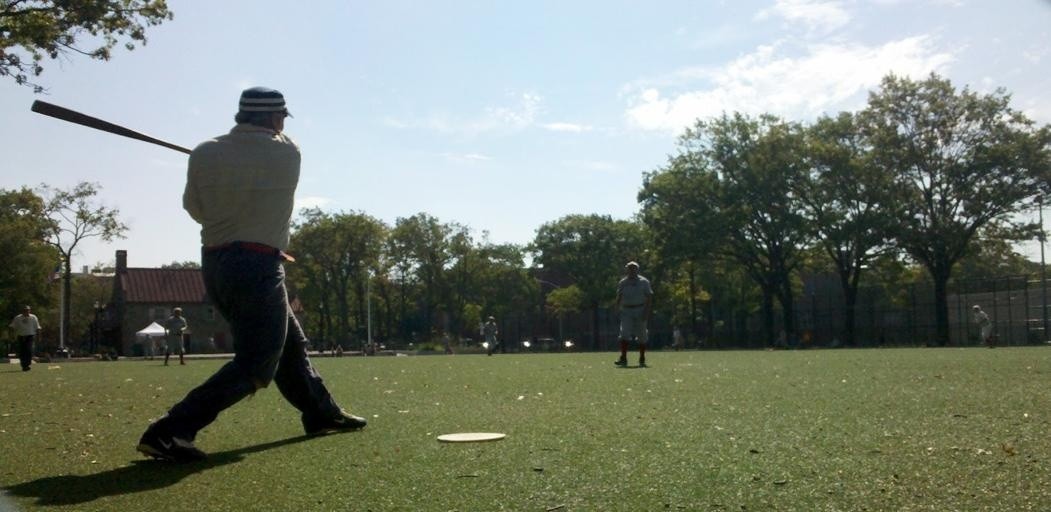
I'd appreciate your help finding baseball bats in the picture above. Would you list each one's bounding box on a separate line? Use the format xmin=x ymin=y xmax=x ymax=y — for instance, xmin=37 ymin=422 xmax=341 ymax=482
xmin=31 ymin=100 xmax=192 ymax=155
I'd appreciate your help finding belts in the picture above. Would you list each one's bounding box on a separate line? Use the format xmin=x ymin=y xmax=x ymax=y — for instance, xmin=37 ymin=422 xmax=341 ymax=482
xmin=239 ymin=242 xmax=295 ymax=261
xmin=626 ymin=305 xmax=641 ymax=308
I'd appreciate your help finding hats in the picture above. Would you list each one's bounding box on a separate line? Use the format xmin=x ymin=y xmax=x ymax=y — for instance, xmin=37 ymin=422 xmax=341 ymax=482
xmin=238 ymin=87 xmax=294 ymax=118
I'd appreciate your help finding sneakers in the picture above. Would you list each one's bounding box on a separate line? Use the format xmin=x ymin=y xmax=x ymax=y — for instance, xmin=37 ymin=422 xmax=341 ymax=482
xmin=302 ymin=409 xmax=367 ymax=436
xmin=137 ymin=422 xmax=213 ymax=468
xmin=614 ymin=359 xmax=627 ymax=365
xmin=639 ymin=355 xmax=645 ymax=363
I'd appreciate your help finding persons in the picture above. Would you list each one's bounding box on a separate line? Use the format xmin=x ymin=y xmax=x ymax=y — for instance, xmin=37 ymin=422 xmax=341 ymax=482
xmin=7 ymin=303 xmax=42 ymax=372
xmin=163 ymin=306 xmax=187 ymax=366
xmin=615 ymin=261 xmax=655 ymax=368
xmin=441 ymin=331 xmax=455 ymax=354
xmin=483 ymin=316 xmax=499 ymax=355
xmin=132 ymin=83 xmax=369 ymax=466
xmin=972 ymin=303 xmax=993 ymax=345
xmin=143 ymin=334 xmax=156 ymax=360
